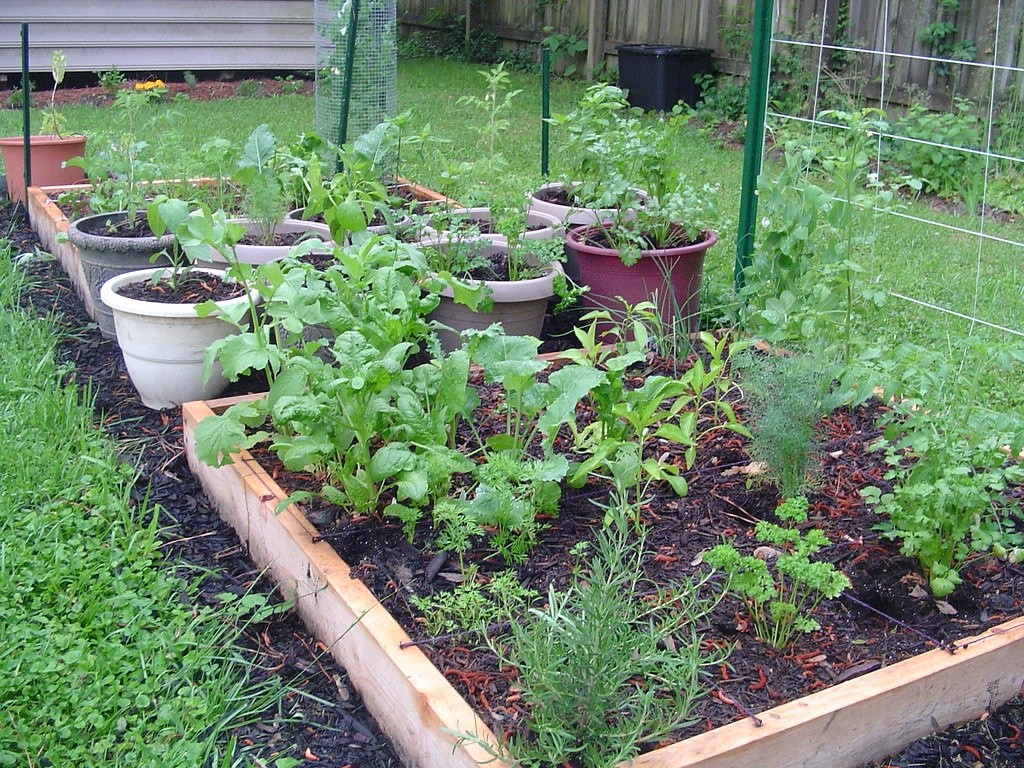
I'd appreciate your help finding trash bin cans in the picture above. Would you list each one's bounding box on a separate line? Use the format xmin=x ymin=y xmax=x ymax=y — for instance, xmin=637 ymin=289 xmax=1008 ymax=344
xmin=614 ymin=42 xmax=717 ymax=118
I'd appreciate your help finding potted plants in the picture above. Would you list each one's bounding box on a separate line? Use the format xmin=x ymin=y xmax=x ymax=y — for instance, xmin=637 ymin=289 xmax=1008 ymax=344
xmin=0 ymin=49 xmax=1024 ymax=767
